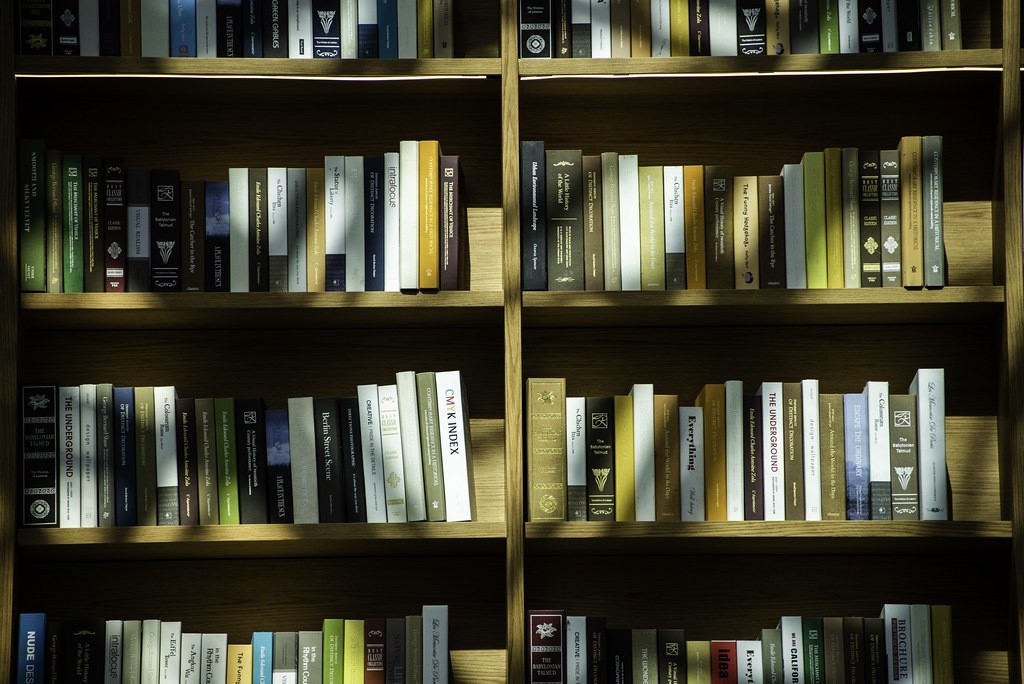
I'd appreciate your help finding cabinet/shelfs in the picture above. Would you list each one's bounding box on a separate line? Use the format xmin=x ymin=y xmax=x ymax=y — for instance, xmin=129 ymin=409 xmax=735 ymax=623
xmin=0 ymin=0 xmax=1024 ymax=684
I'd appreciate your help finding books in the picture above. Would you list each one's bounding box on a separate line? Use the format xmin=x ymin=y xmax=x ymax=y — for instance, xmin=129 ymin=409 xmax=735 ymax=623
xmin=519 ymin=0 xmax=962 ymax=60
xmin=17 ymin=139 xmax=458 ymax=294
xmin=525 ymin=367 xmax=950 ymax=524
xmin=527 ymin=605 xmax=951 ymax=684
xmin=0 ymin=0 xmax=455 ymax=58
xmin=17 ymin=366 xmax=472 ymax=531
xmin=522 ymin=135 xmax=943 ymax=291
xmin=17 ymin=601 xmax=449 ymax=684
